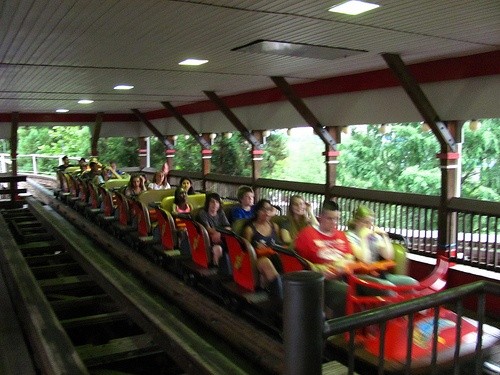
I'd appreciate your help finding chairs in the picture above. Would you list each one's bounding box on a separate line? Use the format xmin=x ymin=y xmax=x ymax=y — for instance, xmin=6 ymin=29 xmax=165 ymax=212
xmin=53 ymin=162 xmax=407 ymax=292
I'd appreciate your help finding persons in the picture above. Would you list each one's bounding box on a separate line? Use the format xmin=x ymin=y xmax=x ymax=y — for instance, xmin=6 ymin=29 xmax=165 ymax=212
xmin=147 ymin=171 xmax=172 ymax=191
xmin=343 ymin=205 xmax=427 ymax=316
xmin=180 ymin=176 xmax=196 ymax=195
xmin=294 ymin=200 xmax=375 ymax=340
xmin=124 ymin=173 xmax=147 ymax=229
xmin=241 ymin=198 xmax=292 ymax=302
xmin=58 ymin=156 xmax=125 ymax=208
xmin=172 ymin=186 xmax=194 ymax=251
xmin=231 ymin=187 xmax=258 ymax=222
xmin=279 ymin=195 xmax=320 ymax=246
xmin=198 ymin=193 xmax=232 ymax=270
